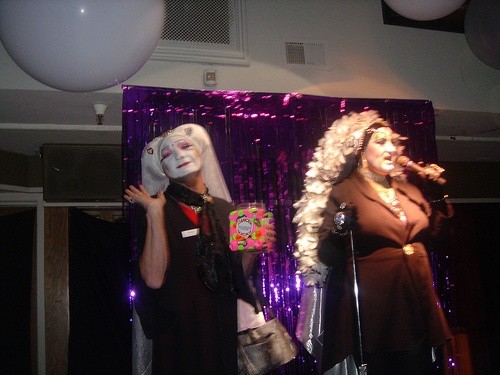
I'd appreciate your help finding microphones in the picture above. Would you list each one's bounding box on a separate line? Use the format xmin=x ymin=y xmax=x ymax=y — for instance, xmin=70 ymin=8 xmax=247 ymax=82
xmin=395 ymin=155 xmax=447 ymax=186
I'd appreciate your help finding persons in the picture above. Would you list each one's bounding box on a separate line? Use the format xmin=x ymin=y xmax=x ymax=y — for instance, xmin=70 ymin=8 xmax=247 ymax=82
xmin=123 ymin=123 xmax=275 ymax=375
xmin=292 ymin=109 xmax=453 ymax=375
